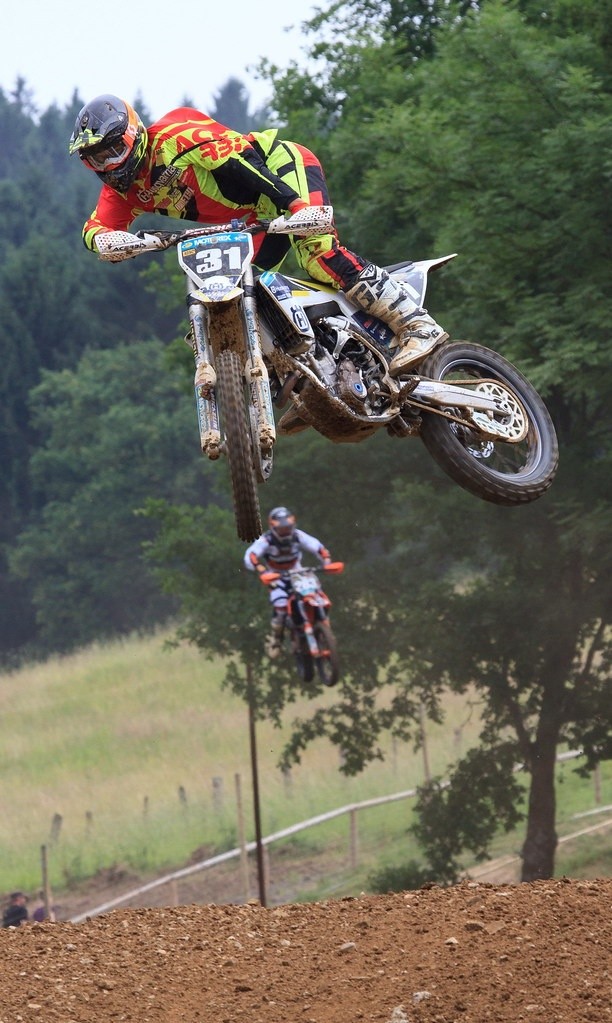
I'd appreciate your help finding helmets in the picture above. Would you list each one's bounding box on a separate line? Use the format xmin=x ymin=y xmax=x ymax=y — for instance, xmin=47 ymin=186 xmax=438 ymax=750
xmin=268 ymin=506 xmax=296 ymax=544
xmin=68 ymin=93 xmax=149 ymax=193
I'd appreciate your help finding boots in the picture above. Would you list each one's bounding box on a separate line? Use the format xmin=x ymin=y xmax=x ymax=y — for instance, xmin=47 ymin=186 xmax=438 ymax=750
xmin=267 ymin=620 xmax=283 ymax=658
xmin=344 ymin=262 xmax=450 ymax=377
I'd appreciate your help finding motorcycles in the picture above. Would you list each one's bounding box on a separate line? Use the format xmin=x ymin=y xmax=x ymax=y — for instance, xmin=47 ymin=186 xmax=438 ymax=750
xmin=93 ymin=204 xmax=562 ymax=544
xmin=260 ymin=561 xmax=345 ymax=687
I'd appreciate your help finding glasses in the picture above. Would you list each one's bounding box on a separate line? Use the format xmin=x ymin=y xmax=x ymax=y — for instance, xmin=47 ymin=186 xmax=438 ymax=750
xmin=79 ymin=136 xmax=133 ymax=174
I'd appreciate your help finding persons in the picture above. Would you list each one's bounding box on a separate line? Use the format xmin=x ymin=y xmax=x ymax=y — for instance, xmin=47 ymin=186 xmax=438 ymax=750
xmin=67 ymin=95 xmax=451 ymax=436
xmin=244 ymin=507 xmax=337 ymax=658
